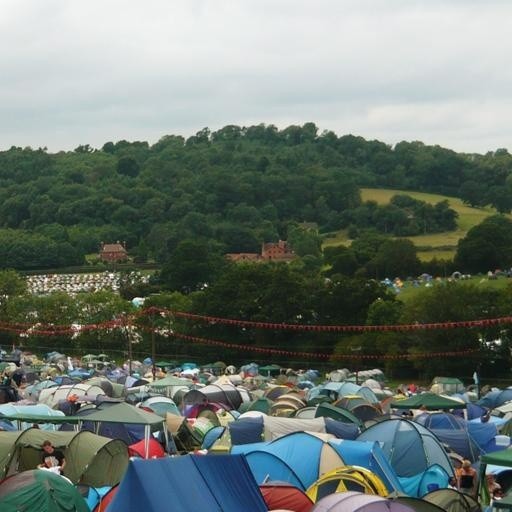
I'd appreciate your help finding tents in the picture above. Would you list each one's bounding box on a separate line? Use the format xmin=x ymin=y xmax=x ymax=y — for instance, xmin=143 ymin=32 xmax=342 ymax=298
xmin=0 ymin=347 xmax=512 ymax=511
